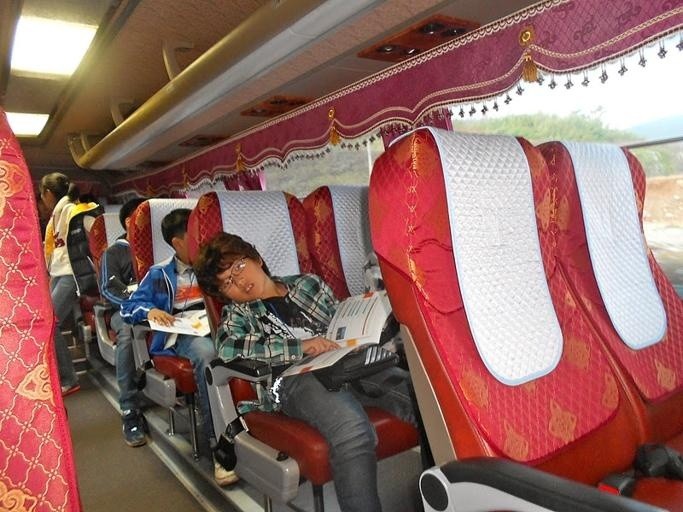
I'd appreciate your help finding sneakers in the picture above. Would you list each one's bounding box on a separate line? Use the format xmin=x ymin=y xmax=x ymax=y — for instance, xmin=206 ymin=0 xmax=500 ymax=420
xmin=121 ymin=409 xmax=146 ymax=447
xmin=61 ymin=384 xmax=80 ymax=397
xmin=212 ymin=451 xmax=240 ymax=486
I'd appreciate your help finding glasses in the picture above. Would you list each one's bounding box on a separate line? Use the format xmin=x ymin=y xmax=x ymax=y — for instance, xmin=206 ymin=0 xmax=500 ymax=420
xmin=217 ymin=256 xmax=248 ymax=294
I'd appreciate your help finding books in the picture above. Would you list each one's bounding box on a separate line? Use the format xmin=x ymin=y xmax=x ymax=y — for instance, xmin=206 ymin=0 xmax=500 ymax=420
xmin=278 ymin=285 xmax=392 ymax=378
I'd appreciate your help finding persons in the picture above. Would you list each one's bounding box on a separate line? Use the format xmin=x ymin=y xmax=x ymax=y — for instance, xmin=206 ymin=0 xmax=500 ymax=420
xmin=29 ymin=170 xmax=240 ymax=490
xmin=192 ymin=224 xmax=425 ymax=512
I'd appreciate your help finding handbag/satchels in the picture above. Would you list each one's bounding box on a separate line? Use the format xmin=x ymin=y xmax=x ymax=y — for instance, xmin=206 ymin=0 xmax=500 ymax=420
xmin=311 ymin=341 xmax=399 ymax=392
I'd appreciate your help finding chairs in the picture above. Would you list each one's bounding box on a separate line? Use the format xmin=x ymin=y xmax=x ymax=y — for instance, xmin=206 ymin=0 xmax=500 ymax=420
xmin=122 ymin=196 xmax=205 ymax=467
xmin=0 ymin=108 xmax=83 ymax=512
xmin=88 ymin=213 xmax=126 ymax=368
xmin=366 ymin=127 xmax=682 ymax=511
xmin=189 ymin=181 xmax=429 ymax=512
xmin=71 ymin=297 xmax=96 ymax=360
